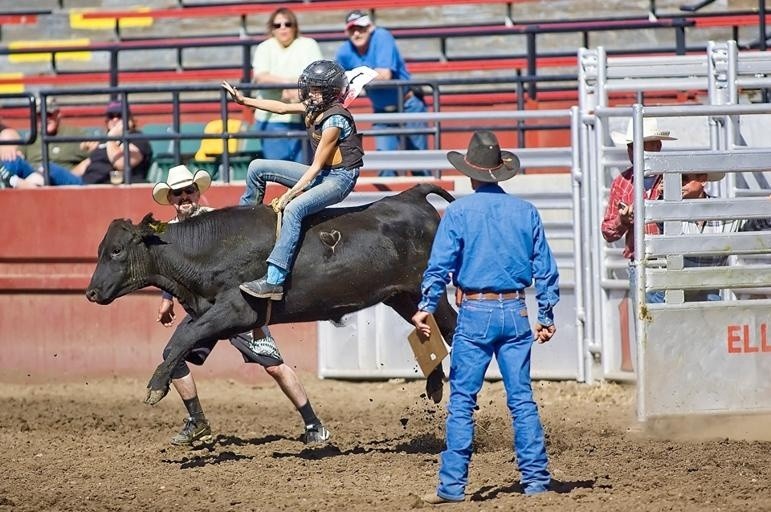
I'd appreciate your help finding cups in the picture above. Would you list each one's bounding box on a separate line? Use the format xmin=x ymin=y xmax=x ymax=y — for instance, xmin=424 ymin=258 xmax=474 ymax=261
xmin=109 ymin=170 xmax=124 ymax=186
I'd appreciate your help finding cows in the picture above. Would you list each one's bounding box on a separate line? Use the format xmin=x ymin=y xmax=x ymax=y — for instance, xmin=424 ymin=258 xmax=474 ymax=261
xmin=85 ymin=184 xmax=459 ymax=406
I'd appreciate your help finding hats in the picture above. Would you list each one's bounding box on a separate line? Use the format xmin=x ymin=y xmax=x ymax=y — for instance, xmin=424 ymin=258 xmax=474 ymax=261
xmin=610 ymin=117 xmax=679 ymax=145
xmin=104 ymin=100 xmax=129 ymax=115
xmin=34 ymin=96 xmax=59 ymax=114
xmin=152 ymin=164 xmax=211 ymax=206
xmin=344 ymin=10 xmax=372 ymax=31
xmin=446 ymin=130 xmax=520 ymax=183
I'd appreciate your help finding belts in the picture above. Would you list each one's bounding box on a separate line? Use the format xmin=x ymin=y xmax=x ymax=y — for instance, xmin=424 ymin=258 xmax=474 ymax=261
xmin=464 ymin=291 xmax=525 ymax=300
xmin=384 ymin=90 xmax=415 ymax=112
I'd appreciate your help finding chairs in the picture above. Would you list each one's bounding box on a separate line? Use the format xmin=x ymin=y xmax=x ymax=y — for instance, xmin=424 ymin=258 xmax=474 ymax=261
xmin=17 ymin=119 xmax=288 ymax=184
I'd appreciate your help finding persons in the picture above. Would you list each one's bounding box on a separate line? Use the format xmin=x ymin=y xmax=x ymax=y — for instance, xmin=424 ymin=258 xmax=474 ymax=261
xmin=601 ymin=118 xmax=677 ymax=308
xmin=645 ymin=173 xmax=769 ymax=303
xmin=220 ymin=60 xmax=364 ymax=302
xmin=251 ymin=6 xmax=322 ymax=165
xmin=412 ymin=131 xmax=560 ymax=503
xmin=334 ymin=10 xmax=435 ymax=177
xmin=151 ymin=162 xmax=333 ymax=448
xmin=0 ymin=101 xmax=152 ymax=189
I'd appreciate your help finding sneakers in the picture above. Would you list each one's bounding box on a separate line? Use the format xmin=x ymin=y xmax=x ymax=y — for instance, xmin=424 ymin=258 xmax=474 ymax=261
xmin=303 ymin=424 xmax=334 ymax=444
xmin=423 ymin=492 xmax=464 ymax=504
xmin=239 ymin=272 xmax=284 ymax=301
xmin=170 ymin=416 xmax=213 ymax=446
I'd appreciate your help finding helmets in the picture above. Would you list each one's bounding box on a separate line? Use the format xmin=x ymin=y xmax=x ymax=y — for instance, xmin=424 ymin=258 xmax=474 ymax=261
xmin=297 ymin=60 xmax=350 ymax=112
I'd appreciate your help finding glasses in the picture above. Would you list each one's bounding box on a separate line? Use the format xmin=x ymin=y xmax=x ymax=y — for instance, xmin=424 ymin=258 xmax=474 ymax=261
xmin=273 ymin=21 xmax=292 ymax=29
